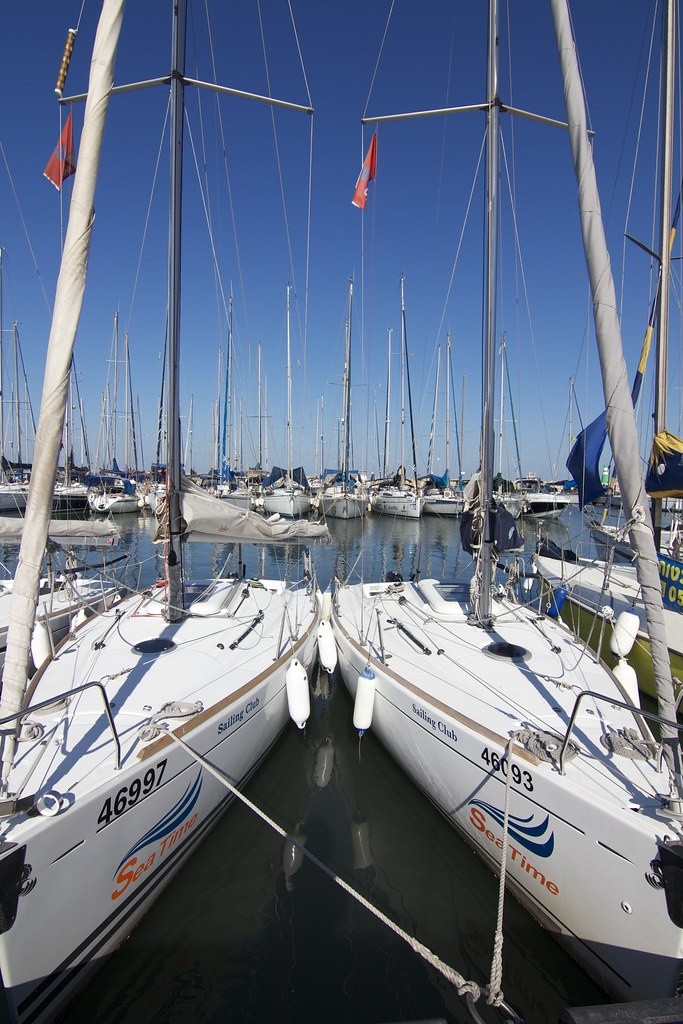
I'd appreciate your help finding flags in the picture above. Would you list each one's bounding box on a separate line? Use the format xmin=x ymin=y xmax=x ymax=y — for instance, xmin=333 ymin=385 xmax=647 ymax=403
xmin=350 ymin=133 xmax=376 ymax=210
xmin=43 ymin=112 xmax=76 ymax=190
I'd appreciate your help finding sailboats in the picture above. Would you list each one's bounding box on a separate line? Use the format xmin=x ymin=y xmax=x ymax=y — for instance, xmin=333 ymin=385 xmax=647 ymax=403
xmin=324 ymin=2 xmax=682 ymax=962
xmin=0 ymin=270 xmax=683 ymax=514
xmin=531 ymin=0 xmax=682 ymax=703
xmin=588 ymin=0 xmax=683 ymax=565
xmin=1 ymin=2 xmax=322 ymax=1024
